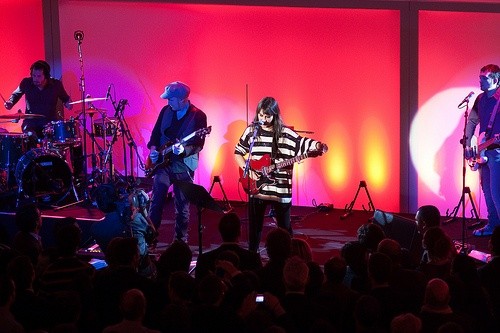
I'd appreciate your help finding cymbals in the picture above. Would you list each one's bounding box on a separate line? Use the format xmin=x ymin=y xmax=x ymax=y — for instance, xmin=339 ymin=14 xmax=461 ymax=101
xmin=69 ymin=97 xmax=108 ymax=105
xmin=0 ymin=112 xmax=47 ymax=119
xmin=74 ymin=107 xmax=110 ymax=114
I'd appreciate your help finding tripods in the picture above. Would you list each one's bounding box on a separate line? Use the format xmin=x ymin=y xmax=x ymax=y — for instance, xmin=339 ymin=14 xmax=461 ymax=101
xmin=51 ymin=94 xmax=127 ymax=212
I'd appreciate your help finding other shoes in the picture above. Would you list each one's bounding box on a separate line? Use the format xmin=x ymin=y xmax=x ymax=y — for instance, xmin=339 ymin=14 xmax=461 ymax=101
xmin=474 ymin=227 xmax=492 ymax=235
xmin=169 ymin=239 xmax=189 ymax=246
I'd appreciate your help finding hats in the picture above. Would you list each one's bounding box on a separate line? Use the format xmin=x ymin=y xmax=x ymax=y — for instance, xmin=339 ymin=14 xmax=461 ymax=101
xmin=160 ymin=82 xmax=190 ymax=99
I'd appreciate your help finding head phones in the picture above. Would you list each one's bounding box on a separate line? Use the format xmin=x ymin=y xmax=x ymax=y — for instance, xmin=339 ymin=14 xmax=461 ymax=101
xmin=39 ymin=62 xmax=51 ymax=79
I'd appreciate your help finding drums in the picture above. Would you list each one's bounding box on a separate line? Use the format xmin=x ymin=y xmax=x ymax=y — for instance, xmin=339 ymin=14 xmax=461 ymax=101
xmin=0 ymin=131 xmax=30 ymax=172
xmin=42 ymin=119 xmax=82 ymax=149
xmin=13 ymin=147 xmax=74 ymax=210
xmin=93 ymin=116 xmax=121 ymax=138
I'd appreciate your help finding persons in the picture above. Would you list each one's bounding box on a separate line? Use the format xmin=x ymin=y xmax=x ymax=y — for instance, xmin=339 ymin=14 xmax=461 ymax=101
xmin=4 ymin=61 xmax=73 ymax=148
xmin=465 ymin=64 xmax=500 ymax=236
xmin=234 ymin=98 xmax=328 ymax=253
xmin=0 ymin=184 xmax=500 ymax=333
xmin=147 ymin=82 xmax=207 ymax=244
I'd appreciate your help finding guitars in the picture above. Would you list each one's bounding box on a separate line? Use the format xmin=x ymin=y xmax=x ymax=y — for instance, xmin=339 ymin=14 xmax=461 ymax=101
xmin=144 ymin=124 xmax=213 ymax=177
xmin=467 ymin=131 xmax=500 ymax=172
xmin=239 ymin=148 xmax=324 ymax=195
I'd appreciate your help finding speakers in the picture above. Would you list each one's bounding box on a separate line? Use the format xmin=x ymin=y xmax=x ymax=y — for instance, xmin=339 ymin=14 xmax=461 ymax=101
xmin=373 ymin=209 xmax=419 ymax=251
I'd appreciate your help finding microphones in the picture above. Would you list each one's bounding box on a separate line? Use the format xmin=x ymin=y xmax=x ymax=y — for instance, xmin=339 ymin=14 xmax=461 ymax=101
xmin=114 ymin=100 xmax=121 ymax=117
xmin=86 ymin=93 xmax=91 ymax=109
xmin=74 ymin=30 xmax=84 ymax=40
xmin=255 ymin=121 xmax=264 ymax=129
xmin=74 ymin=111 xmax=83 ymax=120
xmin=458 ymin=91 xmax=475 ymax=107
xmin=105 ymin=85 xmax=111 ymax=101
